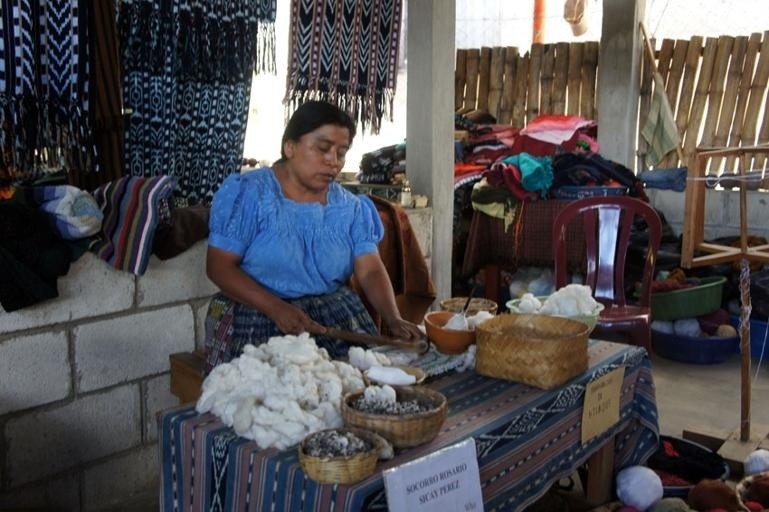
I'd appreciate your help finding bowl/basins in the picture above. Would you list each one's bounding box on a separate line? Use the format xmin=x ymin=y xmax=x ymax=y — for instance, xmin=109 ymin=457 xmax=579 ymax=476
xmin=424 ymin=311 xmax=480 ymax=354
xmin=641 ymin=432 xmax=731 ymax=493
xmin=438 ymin=296 xmax=497 ymax=320
xmin=504 ymin=295 xmax=605 ymax=338
xmin=363 ymin=366 xmax=427 ymax=387
xmin=632 ymin=272 xmax=727 ymax=320
xmin=648 ymin=327 xmax=740 ymax=366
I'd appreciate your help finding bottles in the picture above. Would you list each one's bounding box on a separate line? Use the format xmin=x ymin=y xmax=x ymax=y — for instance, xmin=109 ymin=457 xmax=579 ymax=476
xmin=399 ymin=178 xmax=412 ymax=208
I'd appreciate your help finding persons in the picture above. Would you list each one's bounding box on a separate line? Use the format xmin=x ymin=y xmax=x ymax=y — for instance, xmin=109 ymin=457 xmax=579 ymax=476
xmin=204 ymin=99 xmax=431 ymax=375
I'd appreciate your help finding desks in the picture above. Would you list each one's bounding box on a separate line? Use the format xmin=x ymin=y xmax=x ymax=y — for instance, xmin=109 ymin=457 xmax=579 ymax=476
xmin=154 ymin=318 xmax=646 ymax=512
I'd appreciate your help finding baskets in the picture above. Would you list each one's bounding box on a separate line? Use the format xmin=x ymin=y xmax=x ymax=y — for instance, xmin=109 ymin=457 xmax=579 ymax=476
xmin=440 ymin=296 xmax=497 ymax=316
xmin=298 ymin=428 xmax=382 ymax=485
xmin=341 ymin=387 xmax=447 ymax=447
xmin=475 ymin=313 xmax=588 ymax=391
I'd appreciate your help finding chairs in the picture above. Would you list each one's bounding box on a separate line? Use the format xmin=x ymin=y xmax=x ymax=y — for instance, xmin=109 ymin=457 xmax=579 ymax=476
xmin=551 ymin=196 xmax=664 ymax=355
